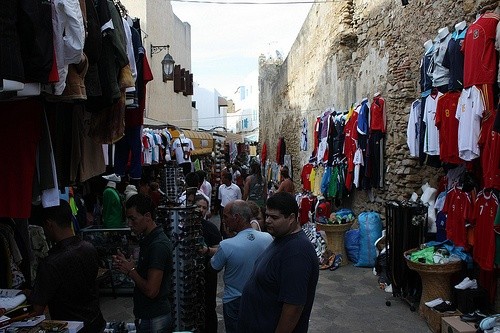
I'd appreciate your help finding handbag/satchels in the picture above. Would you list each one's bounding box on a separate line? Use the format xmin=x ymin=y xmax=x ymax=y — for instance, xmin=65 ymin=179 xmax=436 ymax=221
xmin=183 ymin=150 xmax=190 ymax=160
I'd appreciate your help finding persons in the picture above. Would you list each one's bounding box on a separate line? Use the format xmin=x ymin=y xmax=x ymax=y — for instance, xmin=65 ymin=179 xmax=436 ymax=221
xmin=116 ymin=150 xmax=294 ymax=245
xmin=235 ymin=191 xmax=319 ymax=333
xmin=171 ymin=133 xmax=195 ymax=176
xmin=102 ymin=180 xmax=123 ymax=228
xmin=193 ymin=194 xmax=222 ymax=333
xmin=21 ymin=199 xmax=107 ymax=333
xmin=111 ymin=193 xmax=175 ymax=333
xmin=209 ymin=200 xmax=275 ymax=333
xmin=314 ymin=11 xmax=500 ymax=233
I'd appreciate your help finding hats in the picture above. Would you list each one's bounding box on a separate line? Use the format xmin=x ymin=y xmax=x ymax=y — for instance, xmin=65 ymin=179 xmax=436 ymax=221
xmin=185 ymin=172 xmax=199 ymax=187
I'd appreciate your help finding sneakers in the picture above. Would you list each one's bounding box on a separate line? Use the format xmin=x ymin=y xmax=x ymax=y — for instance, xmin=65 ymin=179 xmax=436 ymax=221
xmin=0 ymin=294 xmax=26 ymax=313
xmin=480 ymin=314 xmax=500 ymax=333
xmin=454 ymin=277 xmax=478 ymax=290
xmin=434 ymin=300 xmax=456 ymax=312
xmin=424 ymin=298 xmax=444 ymax=307
xmin=102 ymin=173 xmax=121 ymax=182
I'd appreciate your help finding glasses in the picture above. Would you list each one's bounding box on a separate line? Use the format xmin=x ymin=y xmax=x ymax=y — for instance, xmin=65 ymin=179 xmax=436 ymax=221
xmin=154 ymin=203 xmax=206 ymax=332
xmin=160 ymin=165 xmax=186 ymax=203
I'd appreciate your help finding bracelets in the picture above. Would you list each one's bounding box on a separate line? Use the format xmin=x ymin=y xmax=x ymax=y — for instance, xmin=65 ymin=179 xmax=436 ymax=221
xmin=128 ymin=267 xmax=136 ymax=275
xmin=205 ymin=248 xmax=210 ymax=254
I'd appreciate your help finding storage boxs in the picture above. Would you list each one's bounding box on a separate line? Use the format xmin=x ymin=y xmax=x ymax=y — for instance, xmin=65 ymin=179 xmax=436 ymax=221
xmin=441 ymin=316 xmax=477 ymax=333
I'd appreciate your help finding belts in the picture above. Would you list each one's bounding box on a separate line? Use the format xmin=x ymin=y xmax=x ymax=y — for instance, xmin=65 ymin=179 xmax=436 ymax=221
xmin=382 ymin=198 xmax=429 ymax=302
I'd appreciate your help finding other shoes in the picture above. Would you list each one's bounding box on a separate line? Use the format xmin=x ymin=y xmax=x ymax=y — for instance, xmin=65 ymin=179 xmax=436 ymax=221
xmin=460 ymin=307 xmax=487 ymax=321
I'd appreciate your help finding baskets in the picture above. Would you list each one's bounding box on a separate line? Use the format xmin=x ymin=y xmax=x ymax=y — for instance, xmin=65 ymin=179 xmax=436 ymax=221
xmin=404 ymin=248 xmax=463 ymax=273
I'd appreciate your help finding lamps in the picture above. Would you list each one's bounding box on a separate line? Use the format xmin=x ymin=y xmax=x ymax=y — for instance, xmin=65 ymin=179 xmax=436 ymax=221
xmin=150 ymin=44 xmax=175 ymax=83
